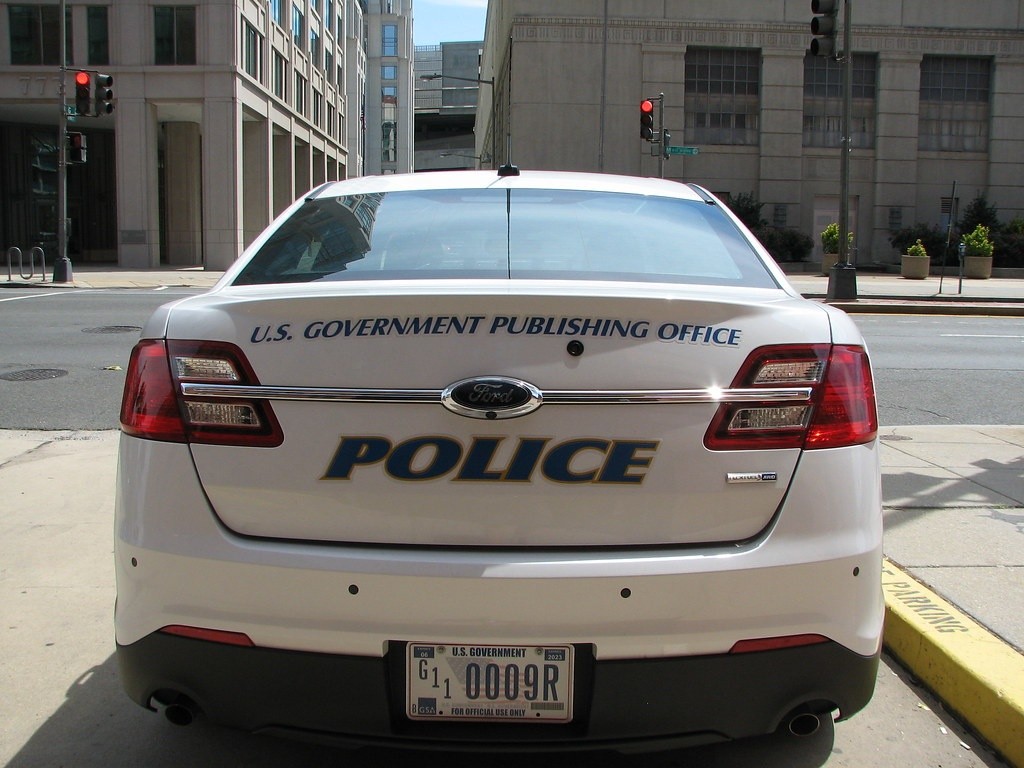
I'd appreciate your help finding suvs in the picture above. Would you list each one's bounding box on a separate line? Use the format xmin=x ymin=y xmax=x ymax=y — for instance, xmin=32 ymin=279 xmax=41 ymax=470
xmin=116 ymin=166 xmax=885 ymax=758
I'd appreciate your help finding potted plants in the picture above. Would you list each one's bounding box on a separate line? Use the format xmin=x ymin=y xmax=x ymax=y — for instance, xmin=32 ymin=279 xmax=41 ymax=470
xmin=901 ymin=238 xmax=929 ymax=279
xmin=820 ymin=222 xmax=856 ymax=276
xmin=959 ymin=224 xmax=993 ymax=279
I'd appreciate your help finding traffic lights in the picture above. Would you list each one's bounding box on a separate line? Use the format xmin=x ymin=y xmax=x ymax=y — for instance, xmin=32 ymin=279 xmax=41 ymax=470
xmin=810 ymin=0 xmax=840 ymax=60
xmin=75 ymin=72 xmax=91 ymax=115
xmin=95 ymin=73 xmax=113 ymax=115
xmin=639 ymin=99 xmax=655 ymax=141
xmin=66 ymin=131 xmax=87 ymax=164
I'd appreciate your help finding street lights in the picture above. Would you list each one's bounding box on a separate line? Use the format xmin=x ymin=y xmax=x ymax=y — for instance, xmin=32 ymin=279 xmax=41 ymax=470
xmin=420 ymin=72 xmax=496 ymax=170
